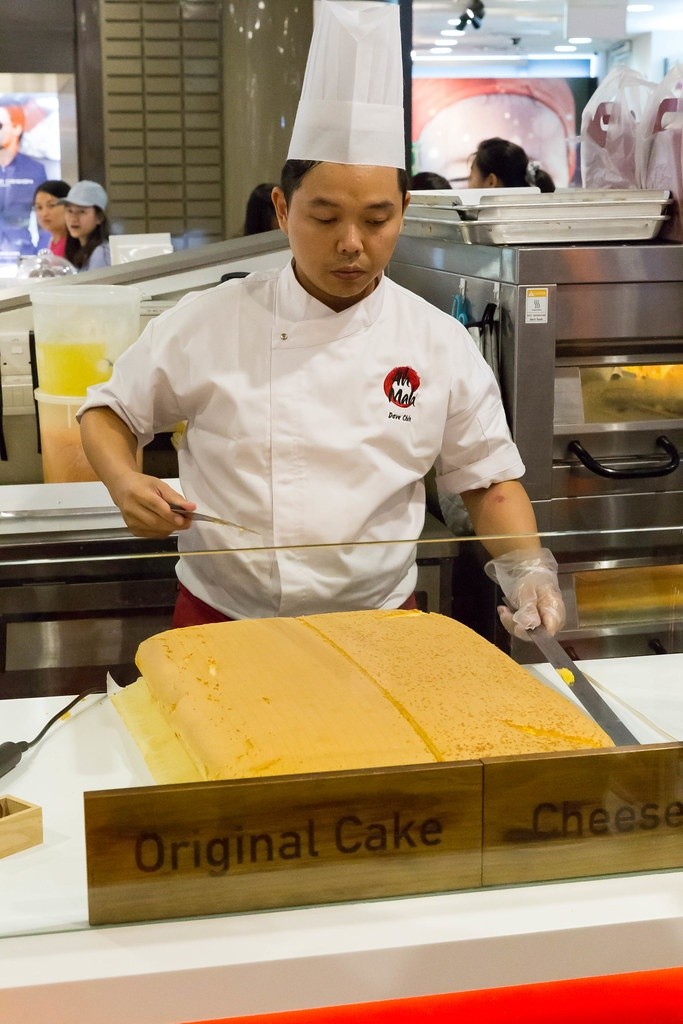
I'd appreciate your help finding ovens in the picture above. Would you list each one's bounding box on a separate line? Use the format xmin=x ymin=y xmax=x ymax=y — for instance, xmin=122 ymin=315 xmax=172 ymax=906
xmin=372 ymin=234 xmax=682 ymax=663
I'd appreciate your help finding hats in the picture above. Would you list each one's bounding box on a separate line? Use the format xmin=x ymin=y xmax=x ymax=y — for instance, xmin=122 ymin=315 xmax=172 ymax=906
xmin=57 ymin=181 xmax=106 ymax=215
xmin=283 ymin=0 xmax=410 ymax=172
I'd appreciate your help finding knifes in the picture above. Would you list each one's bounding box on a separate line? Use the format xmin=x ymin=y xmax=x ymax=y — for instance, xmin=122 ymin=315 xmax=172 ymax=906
xmin=172 ymin=506 xmax=261 ymax=536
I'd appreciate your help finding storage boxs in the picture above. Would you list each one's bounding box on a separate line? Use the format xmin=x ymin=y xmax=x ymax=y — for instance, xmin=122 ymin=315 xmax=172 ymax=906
xmin=30 ymin=284 xmax=141 ymax=397
xmin=34 ymin=387 xmax=144 ymax=484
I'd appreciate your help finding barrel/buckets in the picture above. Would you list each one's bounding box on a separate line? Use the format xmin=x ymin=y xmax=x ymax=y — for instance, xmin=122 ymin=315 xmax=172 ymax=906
xmin=30 ymin=284 xmax=143 ymax=398
xmin=33 ymin=389 xmax=144 ymax=484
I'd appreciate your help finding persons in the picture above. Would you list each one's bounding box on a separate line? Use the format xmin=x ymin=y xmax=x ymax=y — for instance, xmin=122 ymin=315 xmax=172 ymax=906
xmin=75 ymin=1 xmax=568 ymax=642
xmin=53 ymin=180 xmax=110 ymax=271
xmin=0 ymin=98 xmax=47 ymax=266
xmin=241 ymin=139 xmax=555 ymax=236
xmin=34 ymin=177 xmax=70 ymax=260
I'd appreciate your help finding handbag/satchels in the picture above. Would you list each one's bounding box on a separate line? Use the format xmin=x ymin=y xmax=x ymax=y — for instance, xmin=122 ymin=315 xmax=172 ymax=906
xmin=580 ymin=61 xmax=683 ymax=240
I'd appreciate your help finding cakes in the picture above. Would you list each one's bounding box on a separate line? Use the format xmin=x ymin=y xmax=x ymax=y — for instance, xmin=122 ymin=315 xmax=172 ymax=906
xmin=106 ymin=606 xmax=621 ymax=785
